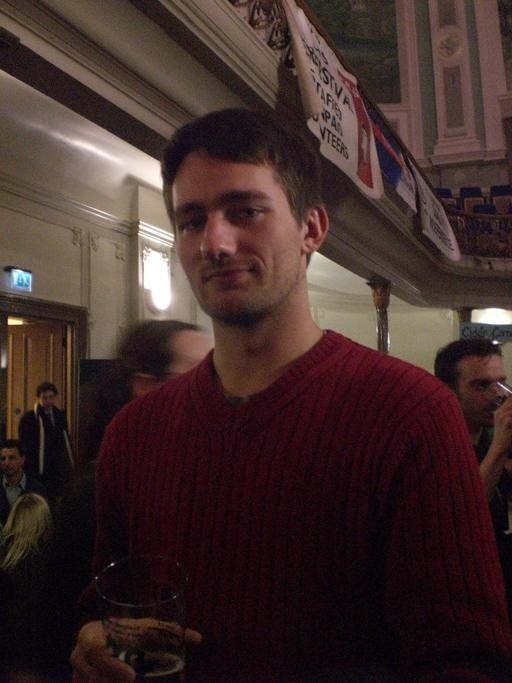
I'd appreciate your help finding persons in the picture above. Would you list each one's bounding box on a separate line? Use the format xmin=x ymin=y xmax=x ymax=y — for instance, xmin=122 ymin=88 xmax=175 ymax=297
xmin=433 ymin=338 xmax=511 ymax=575
xmin=66 ymin=104 xmax=511 ymax=682
xmin=1 ymin=321 xmax=212 ymax=681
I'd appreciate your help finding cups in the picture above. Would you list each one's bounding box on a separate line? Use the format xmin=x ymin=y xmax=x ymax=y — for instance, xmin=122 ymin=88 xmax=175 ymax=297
xmin=94 ymin=552 xmax=193 ymax=683
xmin=484 ymin=381 xmax=511 ymax=427
